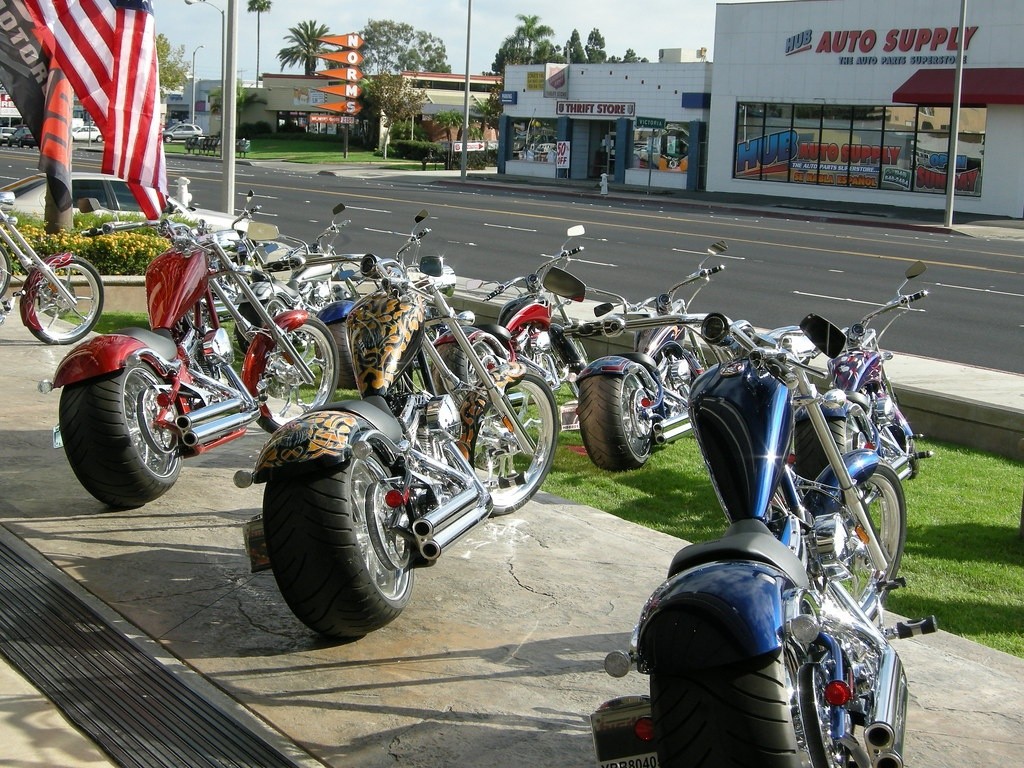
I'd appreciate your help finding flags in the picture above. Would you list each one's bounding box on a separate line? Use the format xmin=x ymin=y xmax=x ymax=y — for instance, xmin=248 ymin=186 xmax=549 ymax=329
xmin=0 ymin=0 xmax=172 ymax=221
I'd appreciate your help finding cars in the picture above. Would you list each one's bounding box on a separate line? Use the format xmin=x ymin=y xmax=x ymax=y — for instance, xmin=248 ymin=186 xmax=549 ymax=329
xmin=0 ymin=126 xmax=19 ymax=147
xmin=513 ymin=134 xmax=558 ymax=162
xmin=162 ymin=123 xmax=204 ymax=142
xmin=0 ymin=170 xmax=253 ymax=243
xmin=6 ymin=123 xmax=40 ymax=150
xmin=634 ymin=133 xmax=690 ymax=171
xmin=72 ymin=126 xmax=103 ymax=143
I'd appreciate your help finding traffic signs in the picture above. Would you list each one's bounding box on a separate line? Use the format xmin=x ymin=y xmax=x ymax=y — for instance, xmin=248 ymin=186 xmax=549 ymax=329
xmin=453 ymin=141 xmax=484 ymax=153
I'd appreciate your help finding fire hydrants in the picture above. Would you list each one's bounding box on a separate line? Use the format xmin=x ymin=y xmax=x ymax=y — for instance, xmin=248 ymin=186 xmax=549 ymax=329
xmin=598 ymin=173 xmax=609 ymax=195
xmin=421 ymin=160 xmax=428 ymax=172
xmin=171 ymin=176 xmax=193 ymax=208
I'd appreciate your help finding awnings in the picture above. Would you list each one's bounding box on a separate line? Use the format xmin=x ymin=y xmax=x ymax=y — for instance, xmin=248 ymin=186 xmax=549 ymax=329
xmin=891 ymin=69 xmax=1024 ymax=104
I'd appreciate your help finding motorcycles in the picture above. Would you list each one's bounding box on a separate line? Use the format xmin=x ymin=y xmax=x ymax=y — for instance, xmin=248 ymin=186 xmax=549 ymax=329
xmin=223 ymin=180 xmax=623 ymax=434
xmin=557 ymin=239 xmax=744 ymax=472
xmin=46 ymin=199 xmax=334 ymax=512
xmin=0 ymin=189 xmax=105 ymax=350
xmin=234 ymin=208 xmax=566 ymax=640
xmin=552 ymin=254 xmax=940 ymax=768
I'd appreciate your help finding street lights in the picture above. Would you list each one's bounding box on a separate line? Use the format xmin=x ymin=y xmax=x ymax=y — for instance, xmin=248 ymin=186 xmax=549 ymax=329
xmin=191 ymin=45 xmax=208 ymax=124
xmin=185 ymin=1 xmax=226 ymax=163
